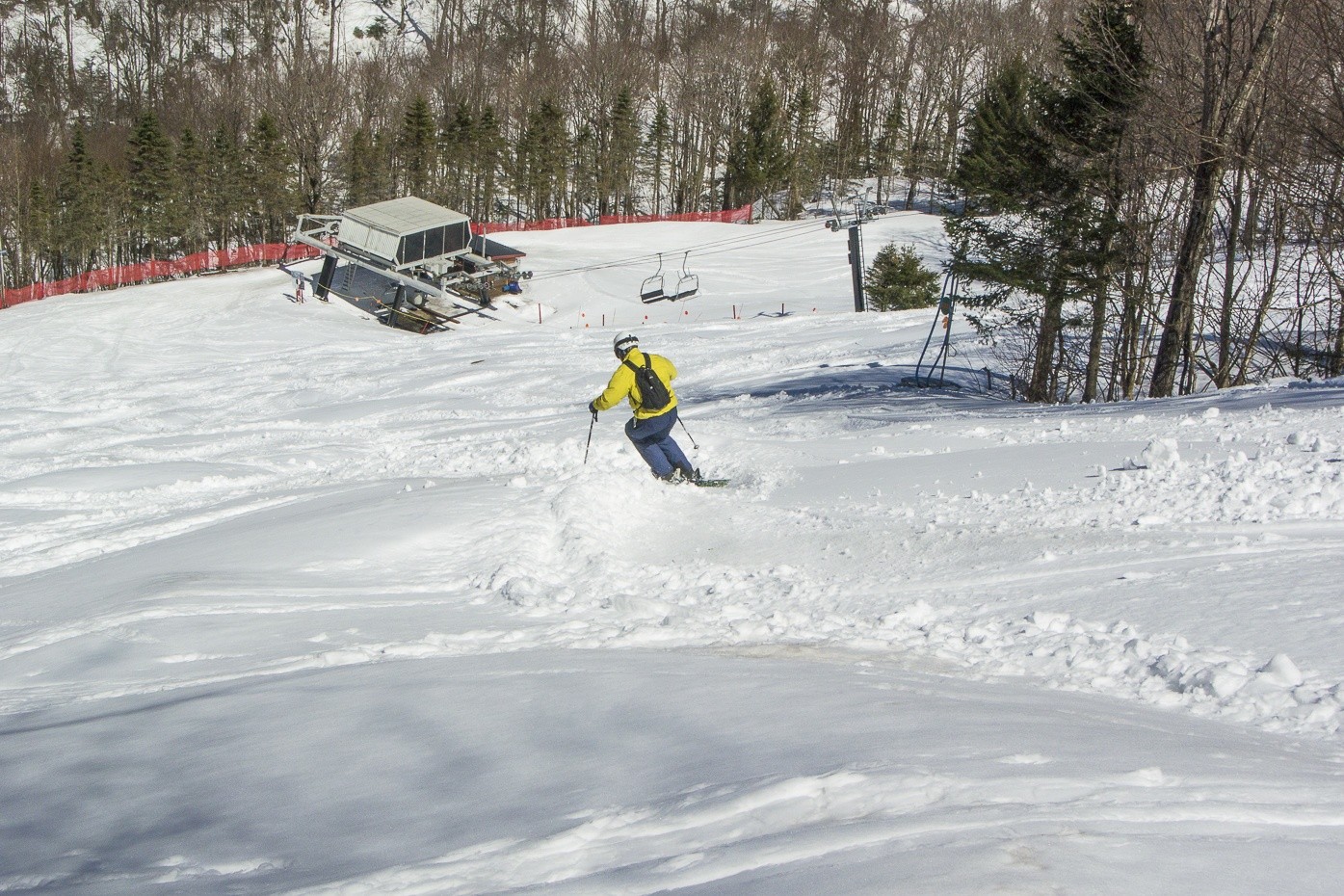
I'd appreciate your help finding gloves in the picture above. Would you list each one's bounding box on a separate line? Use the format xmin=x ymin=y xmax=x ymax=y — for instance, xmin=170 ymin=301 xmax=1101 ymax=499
xmin=588 ymin=402 xmax=598 ymax=414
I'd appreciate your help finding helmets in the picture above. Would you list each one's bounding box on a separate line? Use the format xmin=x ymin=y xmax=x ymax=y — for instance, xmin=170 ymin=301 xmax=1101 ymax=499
xmin=613 ymin=331 xmax=639 ymax=359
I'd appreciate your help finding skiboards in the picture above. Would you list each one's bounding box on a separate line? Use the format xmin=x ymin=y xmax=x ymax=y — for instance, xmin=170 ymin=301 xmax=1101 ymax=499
xmin=683 ymin=477 xmax=732 ymax=488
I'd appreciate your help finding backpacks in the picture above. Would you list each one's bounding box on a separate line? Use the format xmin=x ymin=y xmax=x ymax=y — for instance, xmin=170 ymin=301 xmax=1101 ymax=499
xmin=623 ymin=352 xmax=671 ymax=410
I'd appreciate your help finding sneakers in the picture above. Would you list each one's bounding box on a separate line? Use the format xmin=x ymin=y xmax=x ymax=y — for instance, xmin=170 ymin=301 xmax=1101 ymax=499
xmin=650 ymin=467 xmax=684 ymax=486
xmin=688 ymin=467 xmax=703 ymax=485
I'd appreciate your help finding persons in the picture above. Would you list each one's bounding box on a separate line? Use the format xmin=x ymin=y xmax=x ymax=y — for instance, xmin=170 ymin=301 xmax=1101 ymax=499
xmin=589 ymin=331 xmax=695 ymax=484
